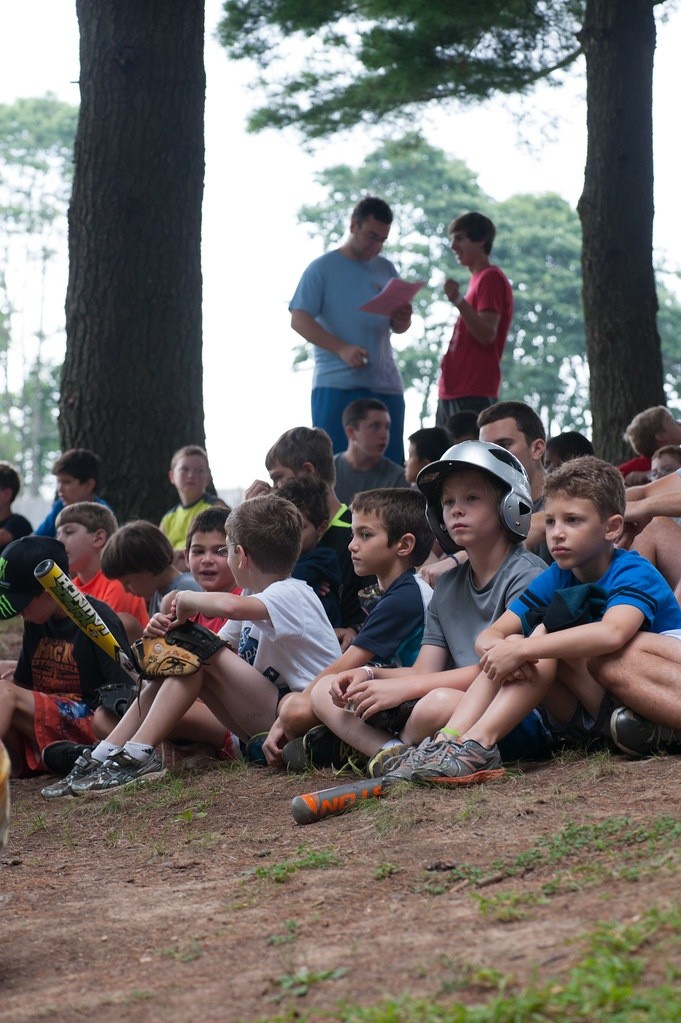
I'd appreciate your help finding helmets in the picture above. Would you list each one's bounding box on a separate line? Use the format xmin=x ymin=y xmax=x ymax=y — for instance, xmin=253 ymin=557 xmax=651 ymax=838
xmin=417 ymin=440 xmax=533 ymax=555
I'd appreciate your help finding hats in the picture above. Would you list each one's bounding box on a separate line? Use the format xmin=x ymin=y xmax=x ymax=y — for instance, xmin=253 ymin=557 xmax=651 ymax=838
xmin=0 ymin=536 xmax=69 ymax=621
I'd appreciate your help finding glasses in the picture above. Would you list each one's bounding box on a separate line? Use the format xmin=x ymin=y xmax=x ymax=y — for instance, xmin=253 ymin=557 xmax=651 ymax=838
xmin=218 ymin=541 xmax=236 ymax=557
xmin=647 ymin=467 xmax=679 ymax=481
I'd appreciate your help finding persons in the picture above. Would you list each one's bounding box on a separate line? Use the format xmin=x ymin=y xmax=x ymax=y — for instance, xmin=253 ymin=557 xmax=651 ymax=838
xmin=32 ymin=448 xmax=115 ymax=538
xmin=101 ymin=520 xmax=205 ymax=645
xmin=0 ymin=536 xmax=132 ymax=776
xmin=274 ymin=477 xmax=341 ymax=627
xmin=328 ymin=440 xmax=551 ymax=789
xmin=437 ymin=212 xmax=513 ymax=438
xmin=55 ymin=501 xmax=149 ymax=632
xmin=266 ymin=425 xmax=376 ymax=652
xmin=310 ymin=440 xmax=548 ymax=777
xmin=92 ymin=509 xmax=247 ymax=762
xmin=287 ymin=194 xmax=405 ymax=466
xmin=0 ymin=514 xmax=32 ymax=553
xmin=404 ymin=427 xmax=450 ymax=492
xmin=40 ymin=496 xmax=342 ymax=799
xmin=0 ymin=462 xmax=20 ymax=520
xmin=258 ymin=488 xmax=433 ymax=768
xmin=448 ymin=401 xmax=681 ymax=758
xmin=332 ymin=398 xmax=409 ymax=508
xmin=154 ymin=444 xmax=228 ymax=572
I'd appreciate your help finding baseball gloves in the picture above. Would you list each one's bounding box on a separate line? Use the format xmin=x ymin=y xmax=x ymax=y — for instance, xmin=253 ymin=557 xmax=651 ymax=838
xmin=93 ymin=682 xmax=138 ymax=720
xmin=129 ymin=616 xmax=235 ymax=719
xmin=357 ymin=583 xmax=384 ymax=616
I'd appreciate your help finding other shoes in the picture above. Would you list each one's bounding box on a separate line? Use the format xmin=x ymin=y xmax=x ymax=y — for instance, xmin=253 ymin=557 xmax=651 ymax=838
xmin=245 ymin=732 xmax=269 ymax=767
xmin=611 ymin=708 xmax=681 ymax=756
xmin=43 ymin=742 xmax=100 ymax=776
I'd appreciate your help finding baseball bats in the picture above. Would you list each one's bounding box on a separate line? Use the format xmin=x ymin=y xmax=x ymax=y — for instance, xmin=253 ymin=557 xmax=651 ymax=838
xmin=290 ymin=775 xmax=386 ymax=826
xmin=34 ymin=558 xmax=148 ymax=688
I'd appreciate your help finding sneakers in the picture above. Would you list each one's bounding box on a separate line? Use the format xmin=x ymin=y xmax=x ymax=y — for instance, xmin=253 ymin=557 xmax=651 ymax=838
xmin=41 ymin=748 xmax=103 ymax=800
xmin=71 ymin=747 xmax=169 ymax=797
xmin=282 ymin=724 xmax=368 ymax=777
xmin=411 ymin=736 xmax=505 ymax=787
xmin=365 ymin=737 xmax=446 ymax=781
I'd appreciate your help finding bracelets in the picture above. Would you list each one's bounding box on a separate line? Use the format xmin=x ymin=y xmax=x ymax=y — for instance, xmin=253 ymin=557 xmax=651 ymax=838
xmin=361 ymin=666 xmax=375 ymax=680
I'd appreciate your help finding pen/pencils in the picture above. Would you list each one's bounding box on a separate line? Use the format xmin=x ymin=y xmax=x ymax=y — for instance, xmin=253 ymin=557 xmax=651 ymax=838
xmin=363 ymin=357 xmax=367 ymax=364
